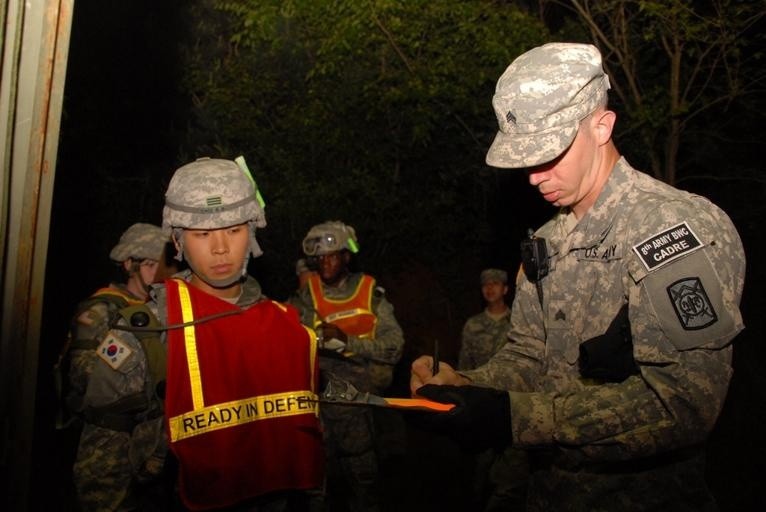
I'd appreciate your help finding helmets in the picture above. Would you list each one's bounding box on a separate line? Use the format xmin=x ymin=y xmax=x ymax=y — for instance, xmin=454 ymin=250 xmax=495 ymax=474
xmin=109 ymin=222 xmax=165 ymax=264
xmin=162 ymin=156 xmax=266 ymax=231
xmin=301 ymin=220 xmax=360 ymax=256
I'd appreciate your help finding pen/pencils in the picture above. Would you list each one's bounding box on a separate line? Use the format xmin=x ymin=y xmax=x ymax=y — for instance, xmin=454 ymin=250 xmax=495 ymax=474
xmin=432 ymin=339 xmax=439 ymax=376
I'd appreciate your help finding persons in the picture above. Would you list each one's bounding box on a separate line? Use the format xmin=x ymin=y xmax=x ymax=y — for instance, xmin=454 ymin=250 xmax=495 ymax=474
xmin=72 ymin=151 xmax=330 ymax=511
xmin=279 ymin=222 xmax=408 ymax=511
xmin=409 ymin=41 xmax=748 ymax=509
xmin=50 ymin=223 xmax=173 ymax=507
xmin=452 ymin=265 xmax=517 ymax=378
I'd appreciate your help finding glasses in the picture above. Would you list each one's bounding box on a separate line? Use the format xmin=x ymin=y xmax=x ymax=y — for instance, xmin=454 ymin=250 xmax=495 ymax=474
xmin=303 ymin=234 xmax=340 ymax=256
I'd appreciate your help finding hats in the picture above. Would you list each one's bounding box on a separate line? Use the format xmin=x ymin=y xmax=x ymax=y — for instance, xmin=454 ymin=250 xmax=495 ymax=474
xmin=479 ymin=268 xmax=509 ymax=284
xmin=485 ymin=42 xmax=612 ymax=169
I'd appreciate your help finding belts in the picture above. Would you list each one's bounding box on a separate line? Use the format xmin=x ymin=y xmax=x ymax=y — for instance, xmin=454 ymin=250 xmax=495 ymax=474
xmin=527 ymin=440 xmax=706 ymax=475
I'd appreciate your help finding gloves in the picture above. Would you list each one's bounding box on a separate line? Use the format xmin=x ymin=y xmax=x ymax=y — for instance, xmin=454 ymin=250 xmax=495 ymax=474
xmin=404 ymin=385 xmax=514 ymax=442
